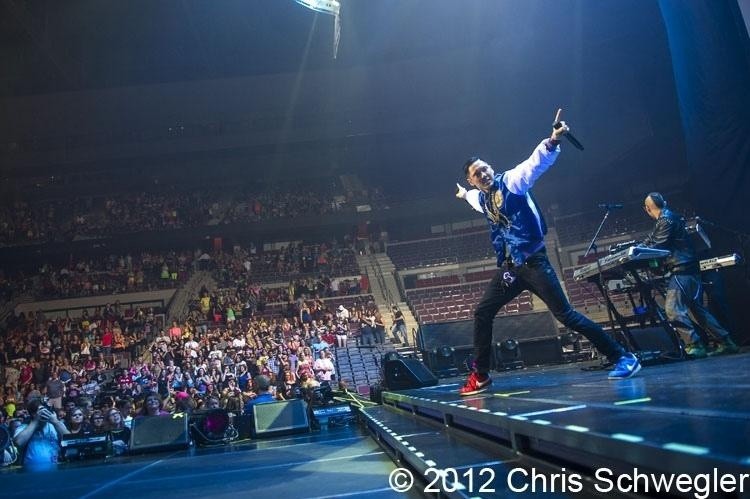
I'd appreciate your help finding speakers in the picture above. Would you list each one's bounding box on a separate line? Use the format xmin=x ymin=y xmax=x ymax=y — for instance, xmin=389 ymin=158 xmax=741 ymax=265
xmin=607 ymin=324 xmax=679 ymax=354
xmin=385 ymin=357 xmax=438 ymax=391
xmin=252 ymin=398 xmax=309 ymax=439
xmin=128 ymin=411 xmax=190 ymax=451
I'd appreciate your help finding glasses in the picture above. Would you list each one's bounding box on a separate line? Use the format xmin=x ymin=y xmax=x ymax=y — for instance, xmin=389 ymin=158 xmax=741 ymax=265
xmin=72 ymin=412 xmax=119 ymax=419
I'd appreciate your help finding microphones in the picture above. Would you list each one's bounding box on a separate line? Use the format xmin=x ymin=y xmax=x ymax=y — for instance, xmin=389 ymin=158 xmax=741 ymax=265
xmin=553 ymin=121 xmax=586 ymax=151
xmin=695 ymin=216 xmax=704 ymax=225
xmin=598 ymin=204 xmax=623 ymax=210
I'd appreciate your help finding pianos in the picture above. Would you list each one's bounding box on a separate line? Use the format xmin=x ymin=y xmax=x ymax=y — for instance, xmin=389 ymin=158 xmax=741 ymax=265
xmin=608 ymin=279 xmax=655 ymax=294
xmin=573 ymin=246 xmax=672 ymax=283
xmin=622 ymin=253 xmax=741 ymax=285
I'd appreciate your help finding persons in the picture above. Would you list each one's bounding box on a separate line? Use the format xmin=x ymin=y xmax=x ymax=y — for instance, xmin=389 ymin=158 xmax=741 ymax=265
xmin=0 ymin=168 xmax=386 ymax=473
xmin=638 ymin=192 xmax=740 ymax=359
xmin=455 ymin=107 xmax=643 ymax=397
xmin=390 ymin=306 xmax=410 ymax=347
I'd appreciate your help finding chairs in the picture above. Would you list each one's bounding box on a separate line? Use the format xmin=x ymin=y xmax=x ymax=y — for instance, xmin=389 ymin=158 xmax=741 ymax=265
xmin=0 ymin=195 xmax=693 ymax=395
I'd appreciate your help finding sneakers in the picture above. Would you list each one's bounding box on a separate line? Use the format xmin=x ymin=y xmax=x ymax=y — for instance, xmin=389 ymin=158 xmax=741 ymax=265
xmin=684 ymin=343 xmax=707 ymax=358
xmin=707 ymin=336 xmax=738 ymax=356
xmin=608 ymin=352 xmax=642 ymax=380
xmin=459 ymin=371 xmax=495 ymax=396
